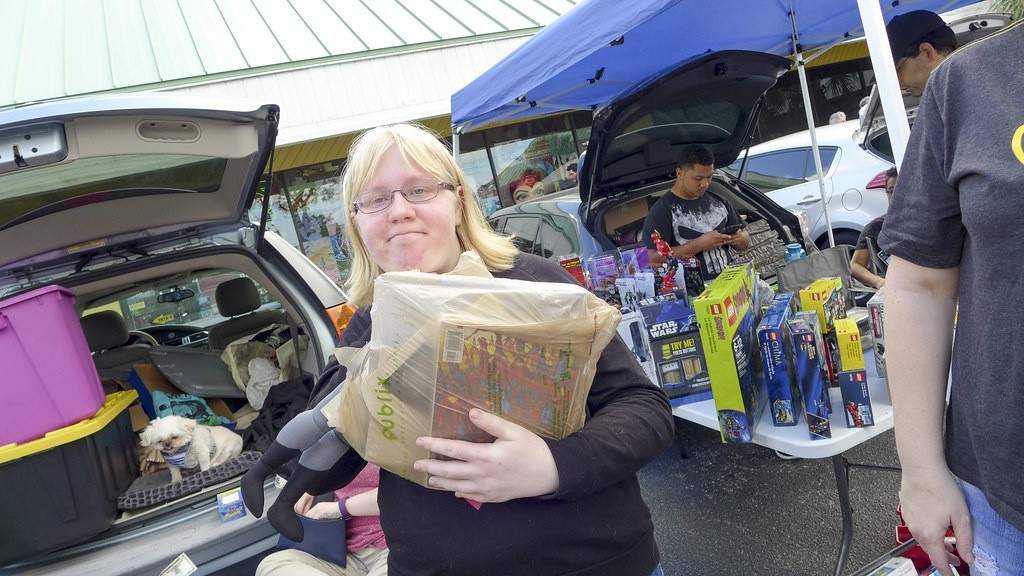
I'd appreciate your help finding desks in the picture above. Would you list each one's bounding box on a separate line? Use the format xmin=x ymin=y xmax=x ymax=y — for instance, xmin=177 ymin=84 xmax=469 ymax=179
xmin=665 ymin=347 xmax=951 ymax=575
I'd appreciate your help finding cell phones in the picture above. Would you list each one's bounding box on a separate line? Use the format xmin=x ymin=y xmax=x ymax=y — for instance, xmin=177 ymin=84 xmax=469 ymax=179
xmin=720 ymin=224 xmax=742 ymax=235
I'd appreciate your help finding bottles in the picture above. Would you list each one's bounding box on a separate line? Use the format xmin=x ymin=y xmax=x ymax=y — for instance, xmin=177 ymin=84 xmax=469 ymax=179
xmin=784 ymin=244 xmax=806 ymax=263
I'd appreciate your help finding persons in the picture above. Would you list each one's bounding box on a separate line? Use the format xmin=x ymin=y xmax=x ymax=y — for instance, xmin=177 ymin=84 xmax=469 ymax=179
xmin=296 ymin=125 xmax=674 ymax=576
xmin=884 ymin=16 xmax=1024 ymax=575
xmin=644 ymin=145 xmax=775 ymax=306
xmin=885 ymin=9 xmax=957 ymax=96
xmin=850 ymin=166 xmax=898 ymax=290
xmin=567 ymin=163 xmax=577 ymax=180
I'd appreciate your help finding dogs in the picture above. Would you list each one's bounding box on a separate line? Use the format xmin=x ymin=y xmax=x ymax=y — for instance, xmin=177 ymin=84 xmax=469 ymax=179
xmin=138 ymin=413 xmax=244 ymax=482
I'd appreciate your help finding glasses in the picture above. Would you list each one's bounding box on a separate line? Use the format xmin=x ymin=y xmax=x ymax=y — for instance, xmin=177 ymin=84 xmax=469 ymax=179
xmin=352 ymin=178 xmax=454 ymax=214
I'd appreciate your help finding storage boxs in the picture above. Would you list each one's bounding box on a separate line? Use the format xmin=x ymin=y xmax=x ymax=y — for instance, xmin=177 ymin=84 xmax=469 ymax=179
xmin=127 ymin=362 xmax=239 ymax=438
xmin=0 ymin=269 xmax=142 ymax=567
xmin=611 ymin=262 xmax=885 ymax=444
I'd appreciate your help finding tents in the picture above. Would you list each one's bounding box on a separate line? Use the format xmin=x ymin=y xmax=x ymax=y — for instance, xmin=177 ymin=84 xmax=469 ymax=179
xmin=449 ymin=0 xmax=991 ymax=248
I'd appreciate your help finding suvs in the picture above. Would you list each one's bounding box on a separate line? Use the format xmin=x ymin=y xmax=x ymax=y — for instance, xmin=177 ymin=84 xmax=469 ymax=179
xmin=481 ymin=49 xmax=819 ymax=314
xmin=718 ymin=11 xmax=1013 ymax=306
xmin=0 ymin=91 xmax=362 ymax=576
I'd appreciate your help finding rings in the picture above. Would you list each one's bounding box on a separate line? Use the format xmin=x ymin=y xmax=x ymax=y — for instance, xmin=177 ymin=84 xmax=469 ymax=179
xmin=723 ymin=241 xmax=726 ymax=245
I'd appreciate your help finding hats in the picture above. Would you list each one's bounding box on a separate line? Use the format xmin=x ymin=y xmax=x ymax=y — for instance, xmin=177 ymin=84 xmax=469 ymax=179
xmin=870 ymin=9 xmax=946 ymax=85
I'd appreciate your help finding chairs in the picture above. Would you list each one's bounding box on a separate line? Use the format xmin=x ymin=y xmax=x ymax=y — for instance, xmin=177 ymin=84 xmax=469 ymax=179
xmin=208 ymin=277 xmax=286 ymax=352
xmin=80 ymin=310 xmax=155 ymax=382
